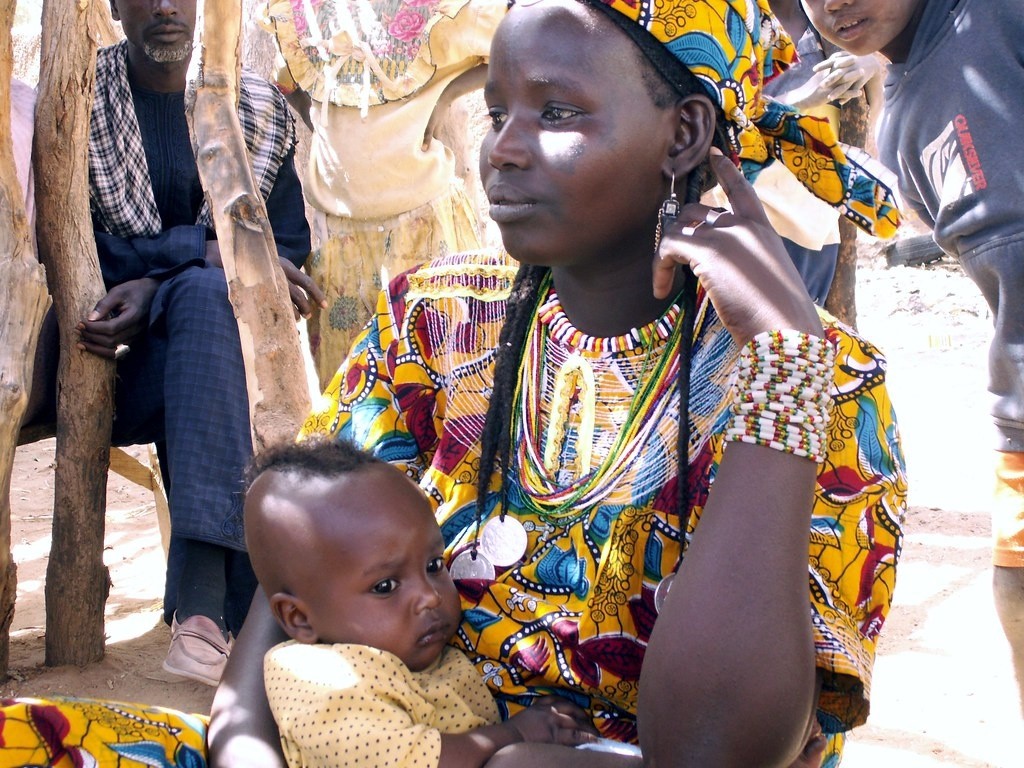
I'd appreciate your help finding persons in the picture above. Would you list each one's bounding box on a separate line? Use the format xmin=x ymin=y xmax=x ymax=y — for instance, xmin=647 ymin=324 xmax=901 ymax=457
xmin=17 ymin=0 xmax=328 ymax=688
xmin=266 ymin=0 xmax=515 ymax=395
xmin=736 ymin=0 xmax=884 ymax=309
xmin=803 ymin=0 xmax=1024 ymax=714
xmin=244 ymin=444 xmax=827 ymax=767
xmin=209 ymin=0 xmax=908 ymax=767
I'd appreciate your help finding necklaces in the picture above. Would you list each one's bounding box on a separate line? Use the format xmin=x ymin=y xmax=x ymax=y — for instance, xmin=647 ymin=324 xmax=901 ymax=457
xmin=510 ymin=267 xmax=736 ymax=525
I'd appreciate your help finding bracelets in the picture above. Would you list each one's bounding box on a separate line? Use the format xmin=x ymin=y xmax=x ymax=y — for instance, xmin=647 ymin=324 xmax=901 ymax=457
xmin=723 ymin=330 xmax=835 ymax=463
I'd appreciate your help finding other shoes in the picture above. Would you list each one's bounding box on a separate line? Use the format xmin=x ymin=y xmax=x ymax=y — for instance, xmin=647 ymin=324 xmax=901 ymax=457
xmin=162 ymin=607 xmax=235 ymax=687
